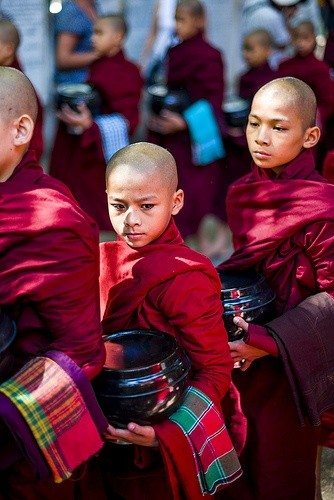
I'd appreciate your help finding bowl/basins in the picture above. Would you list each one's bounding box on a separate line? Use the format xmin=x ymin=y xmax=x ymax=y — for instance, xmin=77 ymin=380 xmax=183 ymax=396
xmin=222 ymin=97 xmax=249 ymax=128
xmin=148 ymin=83 xmax=189 ymax=117
xmin=0 ymin=313 xmax=17 ymax=356
xmin=89 ymin=329 xmax=191 ymax=427
xmin=218 ymin=271 xmax=277 ymax=342
xmin=56 ymin=83 xmax=96 ymax=114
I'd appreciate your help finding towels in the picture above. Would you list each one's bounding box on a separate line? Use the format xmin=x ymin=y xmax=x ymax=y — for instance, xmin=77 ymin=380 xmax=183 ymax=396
xmin=0 ymin=345 xmax=109 ymax=485
xmin=169 ymin=386 xmax=244 ymax=496
xmin=184 ymin=100 xmax=224 ymax=165
xmin=266 ymin=292 xmax=334 ymax=427
xmin=93 ymin=114 xmax=130 ymax=163
